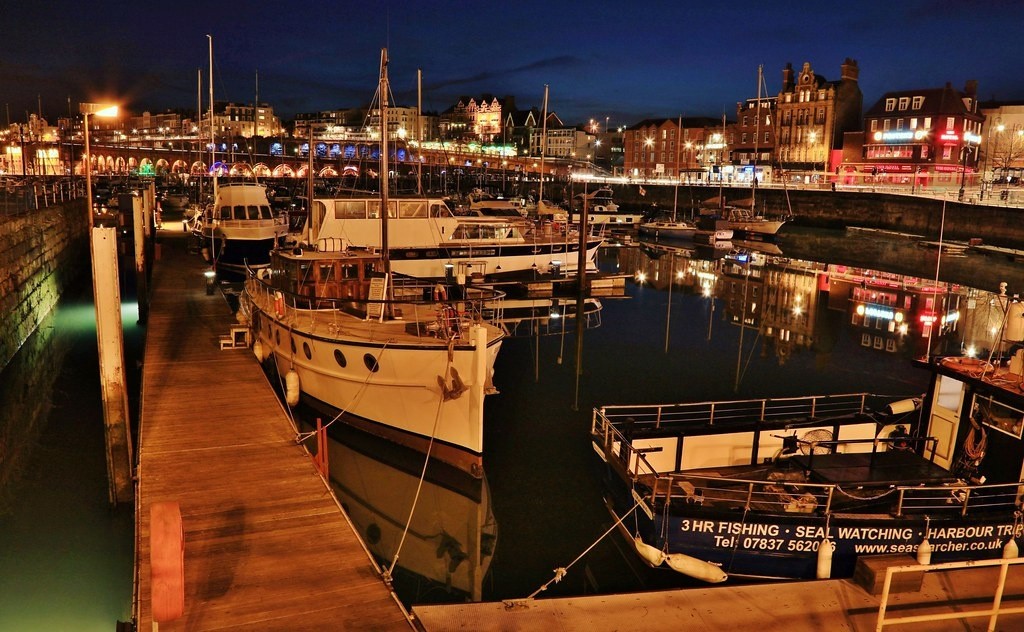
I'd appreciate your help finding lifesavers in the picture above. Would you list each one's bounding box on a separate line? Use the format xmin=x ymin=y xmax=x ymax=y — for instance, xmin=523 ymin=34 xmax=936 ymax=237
xmin=274 ymin=291 xmax=285 ymax=320
xmin=257 ymin=268 xmax=272 ymax=280
xmin=940 ymin=356 xmax=995 ymax=373
xmin=435 ymin=284 xmax=446 ymax=301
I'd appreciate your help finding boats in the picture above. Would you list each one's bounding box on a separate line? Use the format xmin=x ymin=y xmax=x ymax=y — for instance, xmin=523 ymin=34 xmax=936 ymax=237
xmin=564 ymin=188 xmax=644 ymax=226
xmin=590 ymin=343 xmax=1024 ymax=586
xmin=281 ymin=194 xmax=603 ymax=279
xmin=188 ymin=161 xmax=291 ymax=277
xmin=473 ymin=296 xmax=602 ymax=385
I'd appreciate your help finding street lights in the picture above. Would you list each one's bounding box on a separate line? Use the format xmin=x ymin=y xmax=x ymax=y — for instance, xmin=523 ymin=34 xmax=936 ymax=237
xmin=911 ymin=131 xmax=923 ymax=194
xmin=1005 ymin=123 xmax=1023 ymax=196
xmin=959 ymin=132 xmax=972 ymax=203
xmin=80 ymin=101 xmax=118 ymax=315
xmin=808 ymin=130 xmax=818 ymax=174
xmin=394 ymin=129 xmax=406 ymax=197
xmin=980 ymin=116 xmax=1005 ymax=201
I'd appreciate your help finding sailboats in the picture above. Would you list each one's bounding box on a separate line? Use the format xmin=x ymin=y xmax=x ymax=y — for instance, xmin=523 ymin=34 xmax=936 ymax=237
xmin=280 ymin=395 xmax=501 ymax=603
xmin=731 ymin=237 xmax=794 ymax=395
xmin=195 ymin=43 xmax=798 ymax=476
xmin=633 ymin=235 xmax=734 ymax=352
xmin=0 ymin=93 xmax=194 ymax=209
